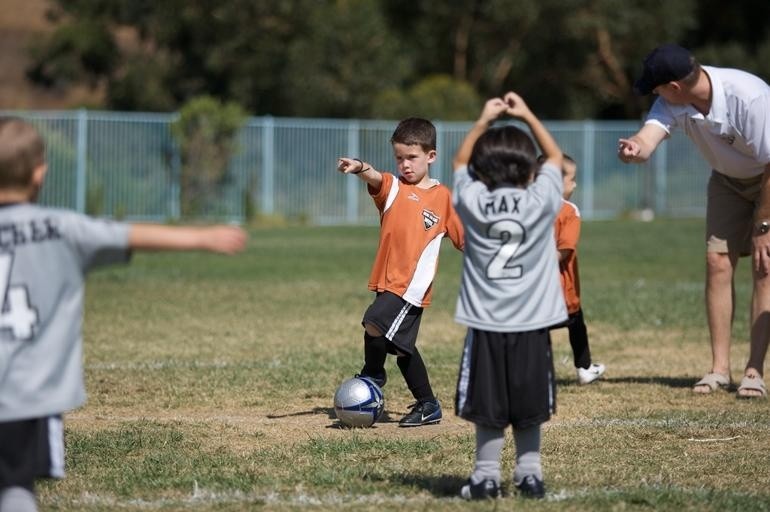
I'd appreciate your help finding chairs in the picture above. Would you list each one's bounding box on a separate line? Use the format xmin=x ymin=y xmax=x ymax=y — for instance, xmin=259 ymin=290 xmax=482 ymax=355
xmin=399 ymin=362 xmax=606 ymax=502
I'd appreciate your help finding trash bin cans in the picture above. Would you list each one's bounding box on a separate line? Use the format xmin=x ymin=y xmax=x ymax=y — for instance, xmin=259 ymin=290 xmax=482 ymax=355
xmin=754 ymin=222 xmax=770 ymax=235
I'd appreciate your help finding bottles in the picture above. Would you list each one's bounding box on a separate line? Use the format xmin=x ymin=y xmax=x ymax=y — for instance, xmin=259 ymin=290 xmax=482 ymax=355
xmin=635 ymin=43 xmax=695 ymax=96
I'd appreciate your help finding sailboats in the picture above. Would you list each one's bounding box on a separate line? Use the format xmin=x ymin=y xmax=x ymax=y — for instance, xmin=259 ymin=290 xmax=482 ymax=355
xmin=335 ymin=379 xmax=383 ymax=426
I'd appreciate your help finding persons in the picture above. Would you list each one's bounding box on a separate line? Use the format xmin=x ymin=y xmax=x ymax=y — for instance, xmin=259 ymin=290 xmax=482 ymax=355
xmin=531 ymin=154 xmax=607 ymax=386
xmin=336 ymin=115 xmax=467 ymax=428
xmin=616 ymin=45 xmax=770 ymax=398
xmin=0 ymin=114 xmax=249 ymax=511
xmin=454 ymin=87 xmax=568 ymax=500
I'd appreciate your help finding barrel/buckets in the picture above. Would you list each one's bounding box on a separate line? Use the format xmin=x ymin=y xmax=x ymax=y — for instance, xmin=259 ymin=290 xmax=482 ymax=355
xmin=737 ymin=376 xmax=766 ymax=398
xmin=692 ymin=372 xmax=733 ymax=394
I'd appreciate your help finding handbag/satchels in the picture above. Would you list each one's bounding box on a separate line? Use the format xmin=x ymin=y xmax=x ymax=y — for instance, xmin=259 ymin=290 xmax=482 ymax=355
xmin=352 ymin=158 xmax=370 ymax=176
